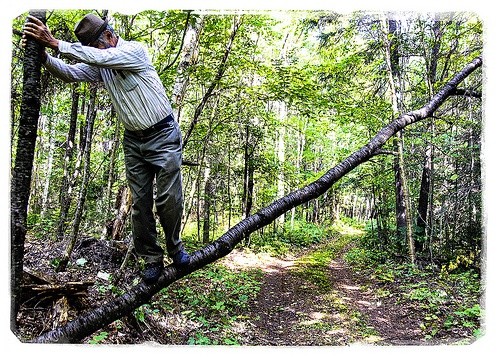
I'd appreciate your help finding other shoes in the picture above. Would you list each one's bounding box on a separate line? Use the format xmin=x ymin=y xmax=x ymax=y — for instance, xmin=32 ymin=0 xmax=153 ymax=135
xmin=143 ymin=261 xmax=164 ymax=282
xmin=170 ymin=248 xmax=191 ymax=264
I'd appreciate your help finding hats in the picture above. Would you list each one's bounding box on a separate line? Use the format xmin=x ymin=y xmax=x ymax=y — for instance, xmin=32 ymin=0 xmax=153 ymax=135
xmin=73 ymin=13 xmax=109 ymax=50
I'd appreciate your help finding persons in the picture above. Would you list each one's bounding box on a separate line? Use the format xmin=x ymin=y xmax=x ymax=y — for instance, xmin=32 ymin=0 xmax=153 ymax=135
xmin=21 ymin=13 xmax=190 ymax=282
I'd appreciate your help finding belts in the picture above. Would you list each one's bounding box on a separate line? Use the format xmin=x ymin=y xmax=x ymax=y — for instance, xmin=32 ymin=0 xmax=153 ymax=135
xmin=122 ymin=113 xmax=176 ymax=137
